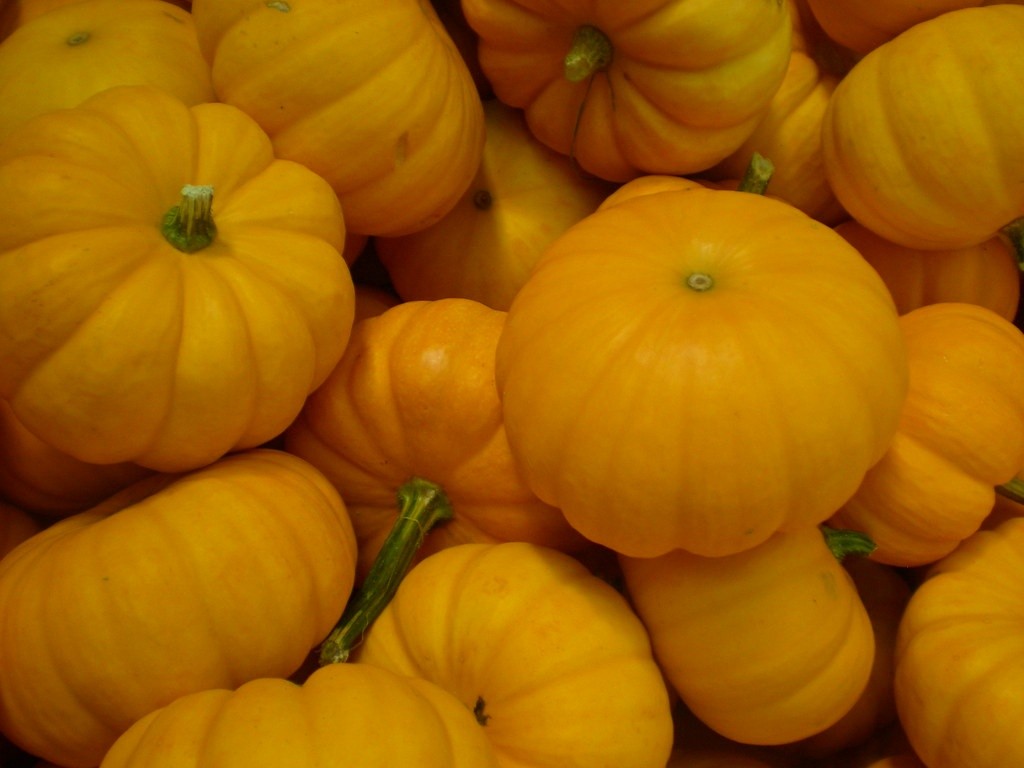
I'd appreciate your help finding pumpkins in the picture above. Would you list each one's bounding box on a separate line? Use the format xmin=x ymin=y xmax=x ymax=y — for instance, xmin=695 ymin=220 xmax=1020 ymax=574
xmin=0 ymin=0 xmax=1024 ymax=768
